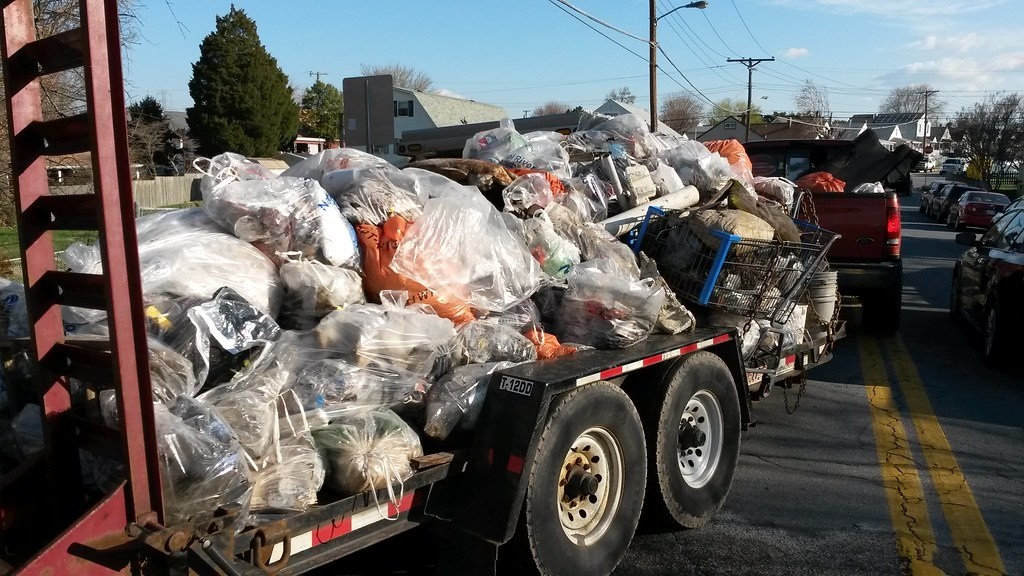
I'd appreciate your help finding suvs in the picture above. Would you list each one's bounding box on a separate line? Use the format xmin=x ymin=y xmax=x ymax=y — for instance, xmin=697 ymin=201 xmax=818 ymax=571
xmin=940 ymin=158 xmax=968 ymax=176
xmin=915 ymin=155 xmax=937 ymax=173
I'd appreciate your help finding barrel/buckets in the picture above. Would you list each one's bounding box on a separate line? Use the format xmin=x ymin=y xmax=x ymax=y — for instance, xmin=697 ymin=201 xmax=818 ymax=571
xmin=789 ymin=271 xmax=837 ymax=355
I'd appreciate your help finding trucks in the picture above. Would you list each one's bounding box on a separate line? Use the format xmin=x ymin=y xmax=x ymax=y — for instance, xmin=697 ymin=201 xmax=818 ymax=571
xmin=0 ymin=0 xmax=903 ymax=576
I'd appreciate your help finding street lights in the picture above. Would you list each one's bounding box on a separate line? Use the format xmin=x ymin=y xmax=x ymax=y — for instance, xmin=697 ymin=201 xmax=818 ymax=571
xmin=649 ymin=1 xmax=708 ymax=136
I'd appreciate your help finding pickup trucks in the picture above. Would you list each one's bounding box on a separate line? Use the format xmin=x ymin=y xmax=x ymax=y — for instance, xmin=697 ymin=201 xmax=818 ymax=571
xmin=736 ymin=139 xmax=903 ymax=336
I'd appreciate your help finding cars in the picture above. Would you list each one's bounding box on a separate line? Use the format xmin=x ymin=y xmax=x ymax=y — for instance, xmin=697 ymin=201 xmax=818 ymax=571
xmin=950 ymin=197 xmax=1024 ymax=363
xmin=947 ymin=191 xmax=1011 ymax=230
xmin=919 ymin=178 xmax=986 ymax=223
